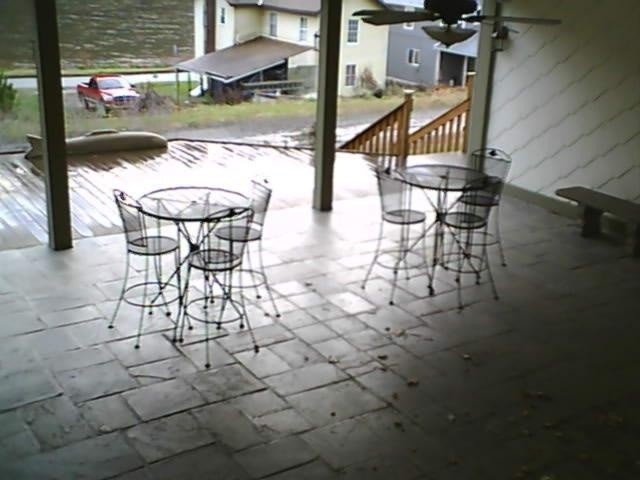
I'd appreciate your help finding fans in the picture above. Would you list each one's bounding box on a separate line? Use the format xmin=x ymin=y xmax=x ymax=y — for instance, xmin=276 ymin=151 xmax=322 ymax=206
xmin=351 ymin=0 xmax=561 ymax=25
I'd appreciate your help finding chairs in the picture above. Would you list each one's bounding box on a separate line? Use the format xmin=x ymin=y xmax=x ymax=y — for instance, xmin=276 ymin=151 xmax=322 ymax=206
xmin=109 ymin=178 xmax=281 ymax=371
xmin=362 ymin=145 xmax=518 ymax=314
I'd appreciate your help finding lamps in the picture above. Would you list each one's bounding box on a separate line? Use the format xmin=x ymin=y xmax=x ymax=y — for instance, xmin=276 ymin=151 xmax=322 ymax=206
xmin=422 ymin=26 xmax=477 ymax=48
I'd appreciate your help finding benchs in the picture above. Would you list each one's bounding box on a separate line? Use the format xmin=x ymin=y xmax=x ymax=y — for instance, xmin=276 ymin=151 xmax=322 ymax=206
xmin=555 ymin=186 xmax=640 ymax=257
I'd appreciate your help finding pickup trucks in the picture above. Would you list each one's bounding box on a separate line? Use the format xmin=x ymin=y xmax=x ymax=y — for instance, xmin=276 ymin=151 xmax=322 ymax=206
xmin=77 ymin=75 xmax=140 ymax=113
